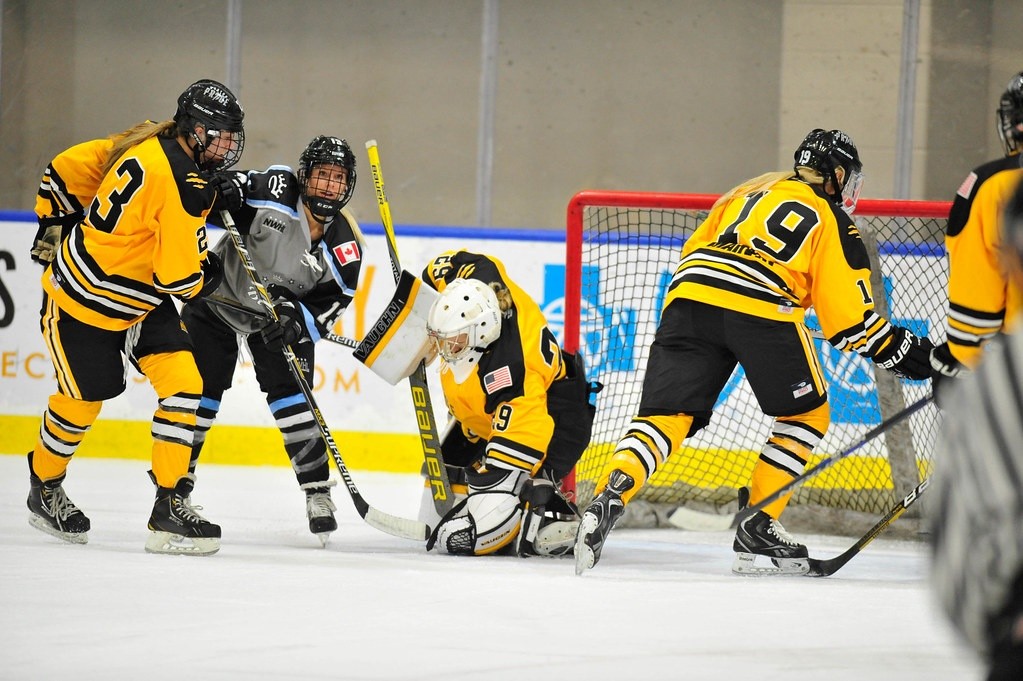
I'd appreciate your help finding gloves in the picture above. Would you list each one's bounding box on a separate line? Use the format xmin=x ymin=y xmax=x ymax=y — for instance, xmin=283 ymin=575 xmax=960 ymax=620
xmin=30 ymin=217 xmax=63 ymax=265
xmin=927 ymin=341 xmax=962 ymax=408
xmin=875 ymin=328 xmax=937 ymax=384
xmin=196 ymin=252 xmax=224 ymax=297
xmin=261 ymin=299 xmax=304 ymax=348
xmin=210 ymin=171 xmax=243 ymax=216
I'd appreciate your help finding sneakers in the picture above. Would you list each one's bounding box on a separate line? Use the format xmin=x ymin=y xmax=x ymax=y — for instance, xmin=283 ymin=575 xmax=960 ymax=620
xmin=27 ymin=450 xmax=91 ymax=544
xmin=534 ymin=491 xmax=583 ymax=557
xmin=731 ymin=487 xmax=809 ymax=575
xmin=575 ymin=470 xmax=635 ymax=577
xmin=305 ymin=487 xmax=338 ymax=549
xmin=147 ymin=470 xmax=222 ymax=554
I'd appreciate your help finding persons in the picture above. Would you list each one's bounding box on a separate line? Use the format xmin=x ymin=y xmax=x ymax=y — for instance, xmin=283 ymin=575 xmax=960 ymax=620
xmin=180 ymin=135 xmax=367 ymax=549
xmin=26 ymin=79 xmax=245 ymax=555
xmin=575 ymin=128 xmax=933 ymax=577
xmin=924 ymin=70 xmax=1023 ymax=681
xmin=351 ymin=248 xmax=597 ymax=558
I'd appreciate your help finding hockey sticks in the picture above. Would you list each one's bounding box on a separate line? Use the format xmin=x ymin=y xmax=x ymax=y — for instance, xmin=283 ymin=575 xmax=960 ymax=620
xmin=202 ymin=293 xmax=363 ymax=348
xmin=218 ymin=208 xmax=431 ymax=541
xmin=770 ymin=474 xmax=933 ymax=578
xmin=667 ymin=392 xmax=934 ymax=531
xmin=364 ymin=138 xmax=456 ymax=519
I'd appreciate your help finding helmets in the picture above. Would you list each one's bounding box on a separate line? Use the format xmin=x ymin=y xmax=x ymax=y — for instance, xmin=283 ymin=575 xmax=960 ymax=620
xmin=296 ymin=136 xmax=358 ymax=217
xmin=793 ymin=129 xmax=864 ymax=215
xmin=996 ymin=73 xmax=1023 ymax=156
xmin=173 ymin=79 xmax=246 ymax=174
xmin=426 ymin=278 xmax=501 ymax=385
